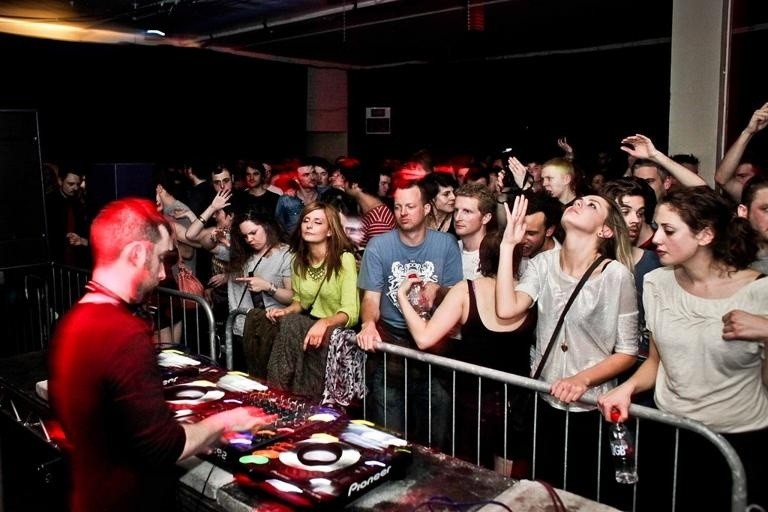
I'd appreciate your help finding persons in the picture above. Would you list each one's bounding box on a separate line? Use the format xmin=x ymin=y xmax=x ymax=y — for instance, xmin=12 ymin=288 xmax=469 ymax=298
xmin=24 ymin=165 xmax=93 ymax=326
xmin=353 ymin=180 xmax=463 ymax=452
xmin=396 ymin=226 xmax=537 ymax=480
xmin=596 ymin=175 xmax=667 ymax=512
xmin=121 ymin=156 xmax=237 ymax=354
xmin=236 ymin=100 xmax=766 ymax=279
xmin=266 ymin=219 xmax=361 ymax=409
xmin=597 ymin=185 xmax=768 ymax=512
xmin=204 ymin=203 xmax=296 ymax=382
xmin=494 ymin=194 xmax=640 ymax=512
xmin=50 ymin=197 xmax=280 ymax=511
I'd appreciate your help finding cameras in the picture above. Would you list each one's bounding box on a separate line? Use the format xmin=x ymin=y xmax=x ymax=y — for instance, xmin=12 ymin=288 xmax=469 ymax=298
xmin=502 ymin=151 xmax=519 ymax=188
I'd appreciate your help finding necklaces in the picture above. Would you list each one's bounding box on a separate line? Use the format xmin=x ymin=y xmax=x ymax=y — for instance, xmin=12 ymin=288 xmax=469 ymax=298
xmin=556 ymin=253 xmax=600 ymax=352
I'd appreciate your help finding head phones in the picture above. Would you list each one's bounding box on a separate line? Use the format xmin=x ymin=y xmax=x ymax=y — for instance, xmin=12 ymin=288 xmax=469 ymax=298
xmin=85 ymin=280 xmax=156 ymax=330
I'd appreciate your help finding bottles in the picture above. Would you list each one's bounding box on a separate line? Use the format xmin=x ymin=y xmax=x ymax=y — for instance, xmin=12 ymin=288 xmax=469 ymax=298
xmin=408 ymin=274 xmax=429 ymax=322
xmin=249 ymin=272 xmax=265 ymax=309
xmin=609 ymin=412 xmax=639 ymax=484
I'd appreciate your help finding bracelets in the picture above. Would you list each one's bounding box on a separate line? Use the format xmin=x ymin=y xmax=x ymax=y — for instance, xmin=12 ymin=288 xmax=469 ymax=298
xmin=264 ymin=282 xmax=278 ymax=297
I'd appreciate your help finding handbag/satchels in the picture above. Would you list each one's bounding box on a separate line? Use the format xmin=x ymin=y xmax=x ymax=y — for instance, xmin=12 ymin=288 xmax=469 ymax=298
xmin=507 ymin=389 xmax=552 ymax=463
xmin=176 ymin=263 xmax=205 ymax=311
